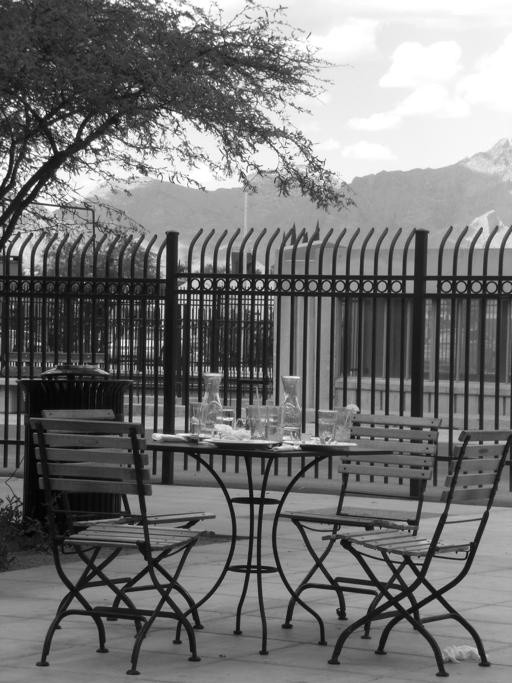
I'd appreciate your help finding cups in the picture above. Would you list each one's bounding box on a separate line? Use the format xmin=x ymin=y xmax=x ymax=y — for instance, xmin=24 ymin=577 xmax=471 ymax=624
xmin=189 ymin=403 xmax=208 ymax=436
xmin=248 ymin=405 xmax=283 ymax=441
xmin=218 ymin=406 xmax=233 ymax=435
xmin=317 ymin=408 xmax=353 ymax=445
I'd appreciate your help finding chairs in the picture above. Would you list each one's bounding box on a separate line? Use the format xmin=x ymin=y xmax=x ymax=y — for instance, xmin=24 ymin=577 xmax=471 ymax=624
xmin=27 ymin=416 xmax=207 ymax=677
xmin=323 ymin=429 xmax=512 ymax=676
xmin=278 ymin=415 xmax=444 ymax=639
xmin=42 ymin=409 xmax=213 ymax=632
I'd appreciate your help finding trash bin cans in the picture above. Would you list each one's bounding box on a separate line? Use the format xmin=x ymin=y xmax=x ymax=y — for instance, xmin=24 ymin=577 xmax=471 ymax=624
xmin=17 ymin=365 xmax=134 ymax=534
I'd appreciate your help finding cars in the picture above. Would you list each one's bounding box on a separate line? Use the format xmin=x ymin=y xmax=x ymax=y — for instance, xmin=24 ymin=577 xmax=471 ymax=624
xmin=111 ymin=325 xmax=186 ymax=368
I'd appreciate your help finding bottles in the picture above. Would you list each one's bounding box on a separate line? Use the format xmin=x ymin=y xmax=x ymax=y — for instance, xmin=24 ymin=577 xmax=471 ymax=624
xmin=199 ymin=373 xmax=222 ymax=438
xmin=281 ymin=375 xmax=302 ymax=442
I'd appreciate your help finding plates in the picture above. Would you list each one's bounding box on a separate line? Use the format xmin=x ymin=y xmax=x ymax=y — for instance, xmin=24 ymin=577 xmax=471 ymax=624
xmin=283 ymin=439 xmax=358 ymax=447
xmin=203 ymin=436 xmax=280 ymax=448
xmin=176 ymin=432 xmax=215 ymax=439
